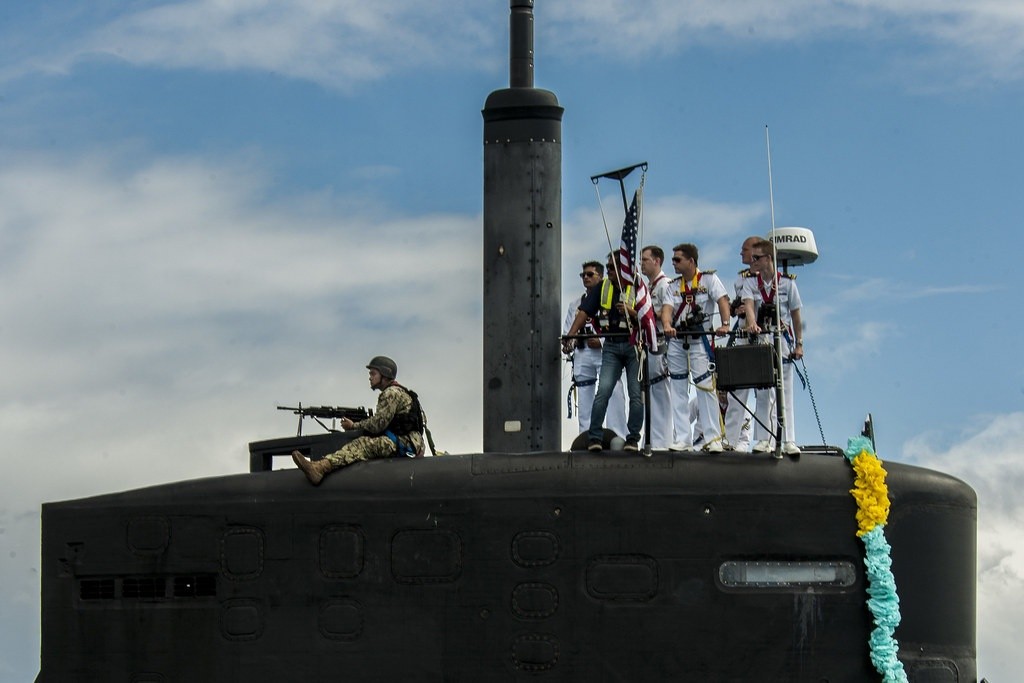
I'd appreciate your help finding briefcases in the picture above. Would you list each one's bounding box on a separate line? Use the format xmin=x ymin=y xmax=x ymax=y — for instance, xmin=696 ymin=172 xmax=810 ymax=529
xmin=715 ymin=338 xmax=776 ymax=391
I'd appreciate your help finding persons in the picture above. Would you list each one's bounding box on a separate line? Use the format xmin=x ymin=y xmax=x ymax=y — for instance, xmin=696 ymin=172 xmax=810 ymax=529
xmin=292 ymin=356 xmax=426 ymax=484
xmin=563 ymin=237 xmax=804 ymax=454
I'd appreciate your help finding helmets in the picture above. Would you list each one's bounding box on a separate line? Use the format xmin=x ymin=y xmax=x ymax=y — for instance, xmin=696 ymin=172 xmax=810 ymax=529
xmin=367 ymin=355 xmax=397 ymax=379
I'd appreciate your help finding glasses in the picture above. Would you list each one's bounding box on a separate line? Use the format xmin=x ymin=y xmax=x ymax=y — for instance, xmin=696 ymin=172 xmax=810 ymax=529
xmin=672 ymin=256 xmax=686 ymax=263
xmin=580 ymin=271 xmax=599 ymax=278
xmin=751 ymin=253 xmax=768 ymax=261
xmin=606 ymin=263 xmax=615 ymax=270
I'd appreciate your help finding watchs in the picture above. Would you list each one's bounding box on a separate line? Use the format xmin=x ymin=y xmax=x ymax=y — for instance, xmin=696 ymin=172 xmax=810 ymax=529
xmin=797 ymin=339 xmax=802 ymax=345
xmin=722 ymin=321 xmax=730 ymax=325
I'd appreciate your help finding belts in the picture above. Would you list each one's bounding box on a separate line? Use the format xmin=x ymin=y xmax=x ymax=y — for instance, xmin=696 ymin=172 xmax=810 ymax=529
xmin=677 ymin=333 xmax=699 ymax=339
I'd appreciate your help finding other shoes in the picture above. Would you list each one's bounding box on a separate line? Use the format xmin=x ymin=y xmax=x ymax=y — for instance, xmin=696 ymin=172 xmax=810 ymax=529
xmin=624 ymin=440 xmax=637 ymax=451
xmin=589 ymin=440 xmax=602 ymax=451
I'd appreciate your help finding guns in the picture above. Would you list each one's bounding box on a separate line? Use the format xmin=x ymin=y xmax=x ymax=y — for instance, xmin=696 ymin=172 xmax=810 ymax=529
xmin=276 ymin=401 xmax=374 ymax=438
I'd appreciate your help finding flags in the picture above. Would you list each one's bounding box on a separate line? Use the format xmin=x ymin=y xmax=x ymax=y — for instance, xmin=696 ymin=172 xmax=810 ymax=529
xmin=620 ymin=189 xmax=658 ymax=352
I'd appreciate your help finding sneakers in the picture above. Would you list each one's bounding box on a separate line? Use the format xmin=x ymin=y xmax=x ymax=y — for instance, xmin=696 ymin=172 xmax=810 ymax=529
xmin=755 ymin=439 xmax=771 ymax=452
xmin=709 ymin=443 xmax=724 ymax=451
xmin=736 ymin=441 xmax=751 ymax=452
xmin=669 ymin=440 xmax=696 ymax=451
xmin=782 ymin=443 xmax=801 ymax=455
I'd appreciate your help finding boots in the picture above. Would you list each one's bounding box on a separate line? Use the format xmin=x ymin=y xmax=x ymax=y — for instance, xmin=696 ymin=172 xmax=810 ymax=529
xmin=292 ymin=449 xmax=330 ymax=483
